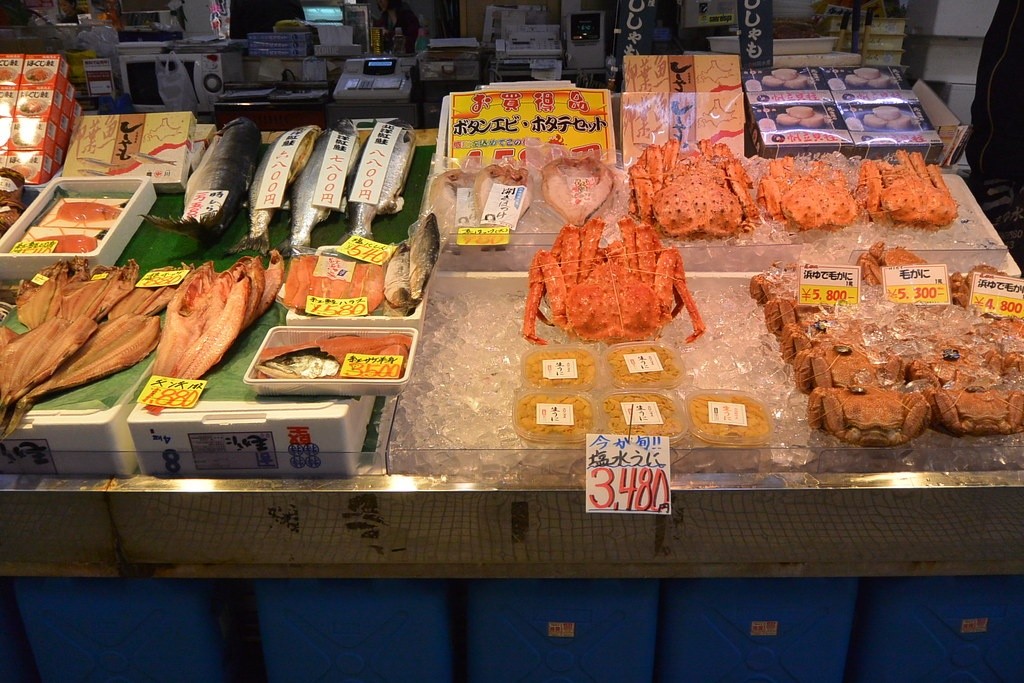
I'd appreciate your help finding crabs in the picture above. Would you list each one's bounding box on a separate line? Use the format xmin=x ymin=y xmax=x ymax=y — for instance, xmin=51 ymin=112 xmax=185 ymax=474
xmin=744 ymin=240 xmax=1024 ymax=449
xmin=626 ymin=137 xmax=762 ymax=241
xmin=521 ymin=216 xmax=706 ymax=346
xmin=756 ymin=148 xmax=959 ymax=233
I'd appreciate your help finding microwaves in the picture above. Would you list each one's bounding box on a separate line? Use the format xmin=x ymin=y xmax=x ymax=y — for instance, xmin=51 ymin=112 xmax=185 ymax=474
xmin=117 ymin=50 xmax=244 ymax=113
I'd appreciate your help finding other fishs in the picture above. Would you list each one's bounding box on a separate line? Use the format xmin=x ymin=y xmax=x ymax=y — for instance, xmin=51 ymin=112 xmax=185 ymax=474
xmin=137 ymin=115 xmax=417 ymax=259
xmin=26 ymin=193 xmax=123 ymax=253
xmin=429 ymin=156 xmax=615 ymax=255
xmin=0 ymin=249 xmax=285 ymax=444
xmin=253 ymin=213 xmax=440 ymax=382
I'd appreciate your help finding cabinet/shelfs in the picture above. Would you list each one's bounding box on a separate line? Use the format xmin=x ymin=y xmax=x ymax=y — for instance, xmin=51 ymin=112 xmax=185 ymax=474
xmin=326 ymin=101 xmax=420 ymax=129
xmin=459 ymin=0 xmax=560 ymax=42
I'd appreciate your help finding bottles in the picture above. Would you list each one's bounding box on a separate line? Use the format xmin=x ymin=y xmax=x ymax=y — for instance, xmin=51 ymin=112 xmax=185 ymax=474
xmin=414 ymin=27 xmax=430 ymax=55
xmin=391 ymin=27 xmax=406 ymax=56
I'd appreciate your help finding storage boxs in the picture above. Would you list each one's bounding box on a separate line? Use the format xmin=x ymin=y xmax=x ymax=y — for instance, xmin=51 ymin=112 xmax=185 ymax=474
xmin=742 ymin=65 xmax=944 ymax=166
xmin=906 ymin=0 xmax=999 ymax=37
xmin=417 ymin=61 xmax=480 ymax=128
xmin=0 ymin=176 xmax=158 ymax=280
xmin=623 ymin=54 xmax=745 ymax=167
xmin=818 ymin=16 xmax=910 ymax=66
xmin=902 ymin=36 xmax=986 ymax=85
xmin=62 ymin=111 xmax=197 ymax=194
xmin=248 ymin=33 xmax=314 ymax=56
xmin=0 ymin=38 xmax=63 ymax=53
xmin=0 ymin=54 xmax=84 ymax=184
xmin=188 ymin=124 xmax=218 ymax=176
xmin=0 ymin=359 xmax=375 ymax=475
xmin=9 ymin=576 xmax=1023 ymax=683
xmin=935 ymin=84 xmax=977 ymax=125
